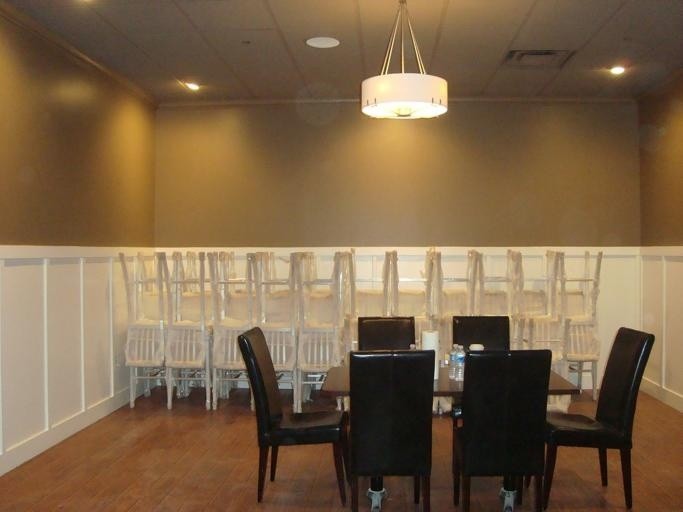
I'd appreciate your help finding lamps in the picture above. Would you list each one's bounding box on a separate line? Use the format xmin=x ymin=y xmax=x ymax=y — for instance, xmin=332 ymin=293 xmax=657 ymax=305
xmin=361 ymin=1 xmax=449 ymax=118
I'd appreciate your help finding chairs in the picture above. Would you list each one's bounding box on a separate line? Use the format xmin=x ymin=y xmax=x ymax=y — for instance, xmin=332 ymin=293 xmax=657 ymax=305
xmin=349 ymin=349 xmax=436 ymax=512
xmin=452 ymin=351 xmax=553 ymax=512
xmin=237 ymin=327 xmax=352 ymax=506
xmin=524 ymin=327 xmax=655 ymax=511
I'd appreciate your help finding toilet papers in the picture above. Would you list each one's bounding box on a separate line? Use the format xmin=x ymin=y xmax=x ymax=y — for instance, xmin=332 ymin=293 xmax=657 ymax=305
xmin=421 ymin=331 xmax=439 ymax=380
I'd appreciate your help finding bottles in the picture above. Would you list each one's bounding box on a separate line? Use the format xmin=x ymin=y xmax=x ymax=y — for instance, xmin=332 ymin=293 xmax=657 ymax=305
xmin=448 ymin=343 xmax=466 ymax=381
